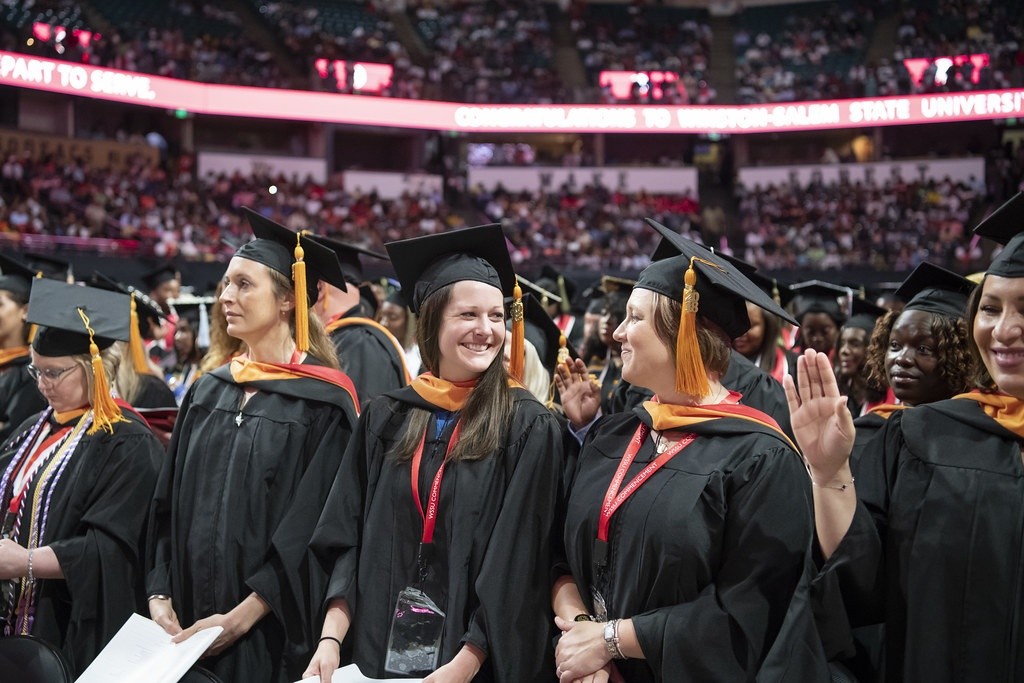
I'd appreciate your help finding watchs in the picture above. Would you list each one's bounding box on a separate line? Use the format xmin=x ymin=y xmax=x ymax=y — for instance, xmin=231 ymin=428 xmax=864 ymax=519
xmin=562 ymin=614 xmax=595 ymax=635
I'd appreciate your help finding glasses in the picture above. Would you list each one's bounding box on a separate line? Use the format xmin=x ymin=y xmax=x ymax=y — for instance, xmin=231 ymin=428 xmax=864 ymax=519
xmin=27 ymin=361 xmax=81 ymax=379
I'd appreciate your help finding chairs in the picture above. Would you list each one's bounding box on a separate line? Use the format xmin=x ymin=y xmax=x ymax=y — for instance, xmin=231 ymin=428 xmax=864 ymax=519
xmin=0 ymin=634 xmax=73 ymax=683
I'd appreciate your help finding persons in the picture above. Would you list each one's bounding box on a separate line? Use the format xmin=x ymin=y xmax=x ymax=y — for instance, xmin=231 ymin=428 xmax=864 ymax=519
xmin=0 ymin=275 xmax=165 ymax=682
xmin=549 ymin=219 xmax=853 ymax=683
xmin=148 ymin=206 xmax=360 ymax=682
xmin=300 ymin=225 xmax=567 ymax=683
xmin=784 ymin=190 xmax=1024 ymax=683
xmin=0 ymin=1 xmax=1024 ymax=444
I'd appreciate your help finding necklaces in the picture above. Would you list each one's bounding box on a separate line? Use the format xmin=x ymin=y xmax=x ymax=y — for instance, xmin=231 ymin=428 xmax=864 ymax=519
xmin=657 ymin=385 xmax=722 ymax=453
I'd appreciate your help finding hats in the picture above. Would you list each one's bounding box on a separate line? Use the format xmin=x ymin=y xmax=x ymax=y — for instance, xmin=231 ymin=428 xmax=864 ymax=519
xmin=144 ymin=264 xmax=176 ymax=289
xmin=601 ymin=275 xmax=635 ymax=313
xmin=631 ymin=217 xmax=801 ymax=397
xmin=502 ymin=292 xmax=582 ymax=399
xmin=87 ymin=269 xmax=166 ymax=376
xmin=752 ymin=272 xmax=797 ymax=313
xmin=897 ymin=263 xmax=978 ymax=318
xmin=975 ymin=192 xmax=1024 ymax=277
xmin=24 ymin=274 xmax=134 ymax=433
xmin=167 ymin=296 xmax=220 ymax=349
xmin=303 ymin=229 xmax=394 ymax=309
xmin=524 ymin=262 xmax=576 ymax=303
xmin=844 ymin=295 xmax=890 ymax=336
xmin=582 ymin=279 xmax=605 ymax=314
xmin=232 ymin=205 xmax=348 ymax=351
xmin=384 ymin=223 xmax=524 ymax=382
xmin=1 ymin=253 xmax=44 ymax=303
xmin=513 ymin=271 xmax=561 ymax=309
xmin=788 ymin=277 xmax=849 ymax=328
xmin=384 ymin=278 xmax=410 ymax=311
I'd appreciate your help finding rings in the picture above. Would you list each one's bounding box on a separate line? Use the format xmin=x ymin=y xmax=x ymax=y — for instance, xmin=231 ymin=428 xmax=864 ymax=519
xmin=558 ymin=666 xmax=563 ymax=674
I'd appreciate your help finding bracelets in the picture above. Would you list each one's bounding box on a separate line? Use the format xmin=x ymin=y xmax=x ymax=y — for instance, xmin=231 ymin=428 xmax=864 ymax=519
xmin=605 ymin=618 xmax=629 ymax=660
xmin=318 ymin=637 xmax=341 ymax=647
xmin=28 ymin=549 xmax=33 ymax=582
xmin=148 ymin=594 xmax=168 ymax=602
xmin=805 ymin=465 xmax=855 ymax=489
